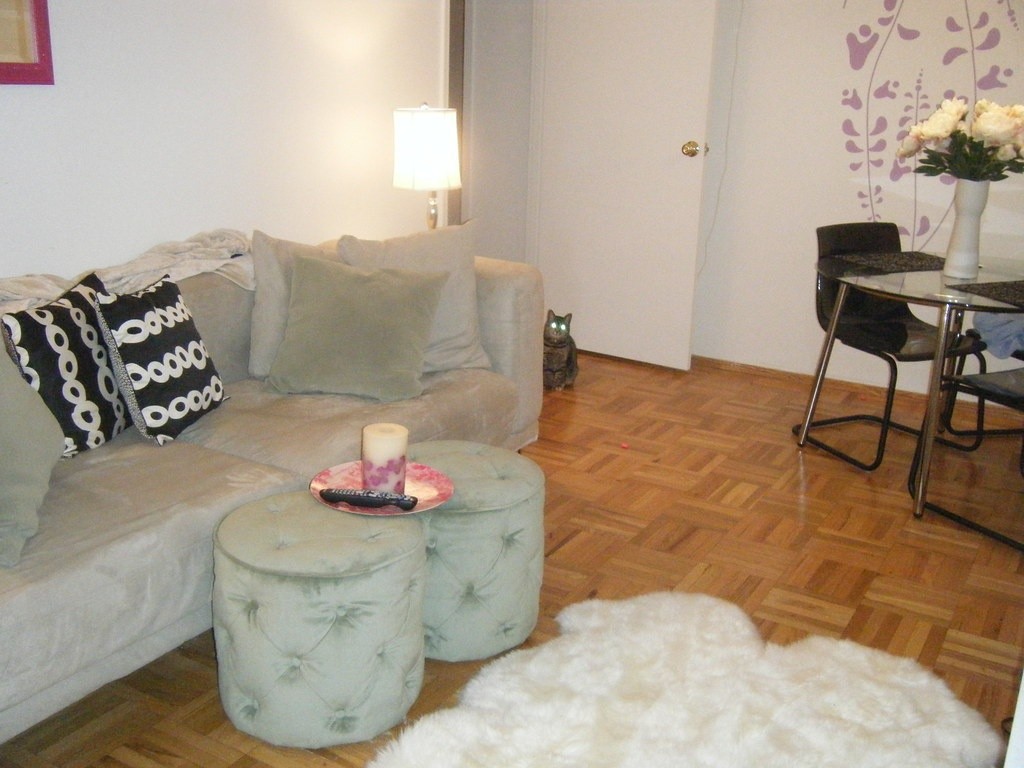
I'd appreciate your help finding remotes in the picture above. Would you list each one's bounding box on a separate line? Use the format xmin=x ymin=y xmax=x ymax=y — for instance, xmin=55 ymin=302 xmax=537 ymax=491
xmin=319 ymin=487 xmax=418 ymax=512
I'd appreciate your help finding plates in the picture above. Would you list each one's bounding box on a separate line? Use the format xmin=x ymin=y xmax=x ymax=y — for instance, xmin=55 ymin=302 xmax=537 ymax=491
xmin=309 ymin=460 xmax=455 ymax=516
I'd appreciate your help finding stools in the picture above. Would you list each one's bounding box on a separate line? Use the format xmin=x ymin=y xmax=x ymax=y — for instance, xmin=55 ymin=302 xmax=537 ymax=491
xmin=214 ymin=492 xmax=426 ymax=746
xmin=394 ymin=439 xmax=546 ymax=664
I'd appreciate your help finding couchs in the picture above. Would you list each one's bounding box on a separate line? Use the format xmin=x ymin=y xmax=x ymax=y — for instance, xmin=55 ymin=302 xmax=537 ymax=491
xmin=0 ymin=224 xmax=542 ymax=749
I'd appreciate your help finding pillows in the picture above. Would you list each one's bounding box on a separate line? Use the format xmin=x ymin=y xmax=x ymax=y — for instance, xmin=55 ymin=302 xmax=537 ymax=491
xmin=248 ymin=222 xmax=493 ymax=399
xmin=0 ymin=273 xmax=231 ymax=560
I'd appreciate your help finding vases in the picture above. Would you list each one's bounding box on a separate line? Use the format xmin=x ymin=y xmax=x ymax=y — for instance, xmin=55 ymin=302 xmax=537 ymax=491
xmin=940 ymin=178 xmax=992 ymax=278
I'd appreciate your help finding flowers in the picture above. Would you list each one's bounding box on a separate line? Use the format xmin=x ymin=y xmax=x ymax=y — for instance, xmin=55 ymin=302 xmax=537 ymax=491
xmin=896 ymin=96 xmax=1024 ymax=182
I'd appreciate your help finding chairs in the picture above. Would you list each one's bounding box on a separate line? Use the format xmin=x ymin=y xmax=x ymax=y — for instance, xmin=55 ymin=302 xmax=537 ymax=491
xmin=791 ymin=222 xmax=1024 ymax=551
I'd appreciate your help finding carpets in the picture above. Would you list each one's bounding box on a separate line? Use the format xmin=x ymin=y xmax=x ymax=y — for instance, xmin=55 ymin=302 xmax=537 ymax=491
xmin=362 ymin=585 xmax=1006 ymax=768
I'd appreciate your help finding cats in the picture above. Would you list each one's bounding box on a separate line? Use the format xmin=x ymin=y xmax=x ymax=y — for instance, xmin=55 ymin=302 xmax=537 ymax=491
xmin=543 ymin=308 xmax=578 ymax=393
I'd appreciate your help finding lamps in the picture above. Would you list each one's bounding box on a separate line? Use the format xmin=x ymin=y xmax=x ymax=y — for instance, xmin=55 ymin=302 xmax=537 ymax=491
xmin=391 ymin=105 xmax=463 ymax=229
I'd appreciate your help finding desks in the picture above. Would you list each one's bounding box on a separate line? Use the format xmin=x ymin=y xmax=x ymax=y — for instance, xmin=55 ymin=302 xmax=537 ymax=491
xmin=795 ymin=251 xmax=1021 ymax=518
